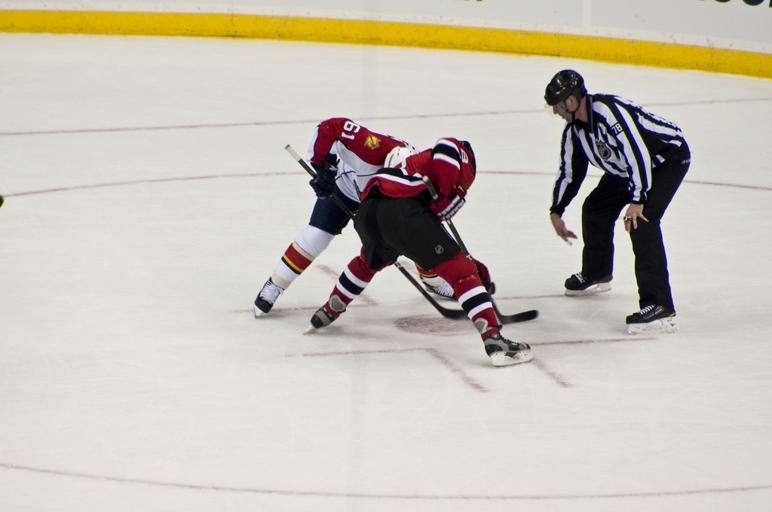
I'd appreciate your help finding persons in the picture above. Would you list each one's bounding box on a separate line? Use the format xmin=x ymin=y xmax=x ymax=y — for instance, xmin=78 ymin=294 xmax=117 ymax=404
xmin=545 ymin=70 xmax=690 ymax=325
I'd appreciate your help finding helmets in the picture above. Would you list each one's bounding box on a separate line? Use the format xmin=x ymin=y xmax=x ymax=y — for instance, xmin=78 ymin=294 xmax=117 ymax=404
xmin=543 ymin=69 xmax=582 ymax=106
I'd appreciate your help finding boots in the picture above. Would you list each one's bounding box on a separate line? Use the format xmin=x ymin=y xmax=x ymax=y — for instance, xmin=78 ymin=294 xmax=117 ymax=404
xmin=564 ymin=271 xmax=613 ymax=290
xmin=626 ymin=303 xmax=677 ymax=324
xmin=310 ymin=302 xmax=340 ymax=329
xmin=422 ymin=276 xmax=454 ymax=299
xmin=254 ymin=275 xmax=285 ymax=314
xmin=482 ymin=334 xmax=531 ymax=356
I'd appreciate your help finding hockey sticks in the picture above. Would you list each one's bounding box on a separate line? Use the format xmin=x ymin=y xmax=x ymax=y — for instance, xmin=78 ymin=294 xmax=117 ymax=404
xmin=285 ymin=142 xmax=467 ymax=320
xmin=422 ymin=172 xmax=539 ymax=324
